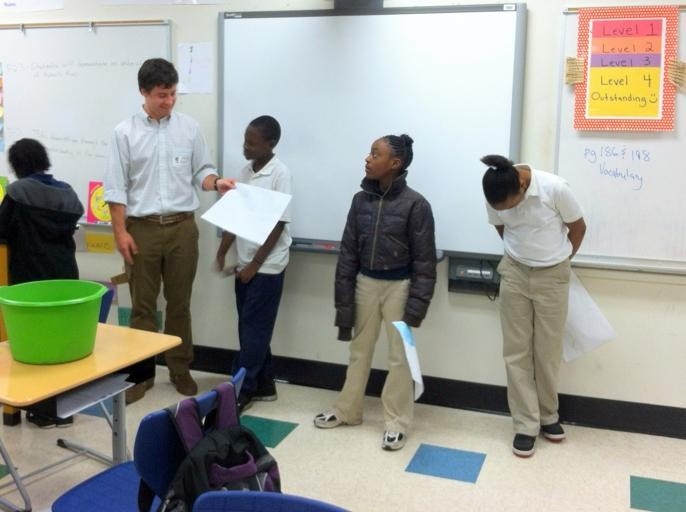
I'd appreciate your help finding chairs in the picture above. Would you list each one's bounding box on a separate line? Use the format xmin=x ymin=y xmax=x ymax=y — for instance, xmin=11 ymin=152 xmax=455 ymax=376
xmin=94 ymin=280 xmax=115 ymax=322
xmin=51 ymin=367 xmax=249 ymax=512
xmin=193 ymin=490 xmax=351 ymax=512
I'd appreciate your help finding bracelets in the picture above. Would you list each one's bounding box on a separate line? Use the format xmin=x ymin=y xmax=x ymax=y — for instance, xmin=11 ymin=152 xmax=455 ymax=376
xmin=253 ymin=255 xmax=265 ymax=266
xmin=214 ymin=178 xmax=219 ymax=191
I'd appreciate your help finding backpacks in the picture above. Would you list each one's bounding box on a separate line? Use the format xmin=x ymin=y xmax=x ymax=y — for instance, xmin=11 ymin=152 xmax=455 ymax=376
xmin=138 ymin=382 xmax=281 ymax=512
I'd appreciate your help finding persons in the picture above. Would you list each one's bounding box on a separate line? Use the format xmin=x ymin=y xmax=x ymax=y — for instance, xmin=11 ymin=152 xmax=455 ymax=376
xmin=105 ymin=57 xmax=236 ymax=406
xmin=0 ymin=138 xmax=85 ymax=428
xmin=480 ymin=156 xmax=586 ymax=458
xmin=216 ymin=114 xmax=292 ymax=415
xmin=313 ymin=134 xmax=437 ymax=451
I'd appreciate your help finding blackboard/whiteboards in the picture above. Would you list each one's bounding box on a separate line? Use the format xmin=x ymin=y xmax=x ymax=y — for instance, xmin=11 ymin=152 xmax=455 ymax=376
xmin=217 ymin=4 xmax=527 ymax=261
xmin=0 ymin=20 xmax=172 ymax=226
xmin=550 ymin=4 xmax=686 ymax=275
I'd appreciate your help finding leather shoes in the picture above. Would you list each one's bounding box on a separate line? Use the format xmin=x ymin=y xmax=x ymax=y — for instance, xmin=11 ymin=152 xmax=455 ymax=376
xmin=126 ymin=377 xmax=154 ymax=405
xmin=170 ymin=372 xmax=198 ymax=395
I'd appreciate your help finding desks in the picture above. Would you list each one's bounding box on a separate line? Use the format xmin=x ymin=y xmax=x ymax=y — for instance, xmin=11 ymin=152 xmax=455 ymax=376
xmin=0 ymin=322 xmax=183 ymax=512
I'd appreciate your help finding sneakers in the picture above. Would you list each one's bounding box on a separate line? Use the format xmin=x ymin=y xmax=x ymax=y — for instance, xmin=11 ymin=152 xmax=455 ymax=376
xmin=53 ymin=410 xmax=74 ymax=428
xmin=252 ymin=380 xmax=277 ymax=401
xmin=541 ymin=422 xmax=566 ymax=440
xmin=382 ymin=431 xmax=405 ymax=451
xmin=237 ymin=389 xmax=253 ymax=412
xmin=315 ymin=411 xmax=348 ymax=428
xmin=26 ymin=410 xmax=56 ymax=429
xmin=513 ymin=433 xmax=535 ymax=456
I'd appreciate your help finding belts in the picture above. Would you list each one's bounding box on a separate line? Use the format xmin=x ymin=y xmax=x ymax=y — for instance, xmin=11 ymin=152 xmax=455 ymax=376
xmin=143 ymin=212 xmax=191 ymax=225
